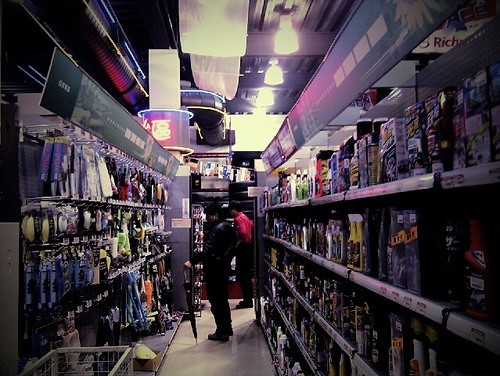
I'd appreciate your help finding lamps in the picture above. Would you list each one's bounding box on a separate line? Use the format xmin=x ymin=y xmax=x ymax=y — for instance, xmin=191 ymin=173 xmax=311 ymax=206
xmin=263 ymin=60 xmax=284 ymax=86
xmin=274 ymin=14 xmax=298 ymax=54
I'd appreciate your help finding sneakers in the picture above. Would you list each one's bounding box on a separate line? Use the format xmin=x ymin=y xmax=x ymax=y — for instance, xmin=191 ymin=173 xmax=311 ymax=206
xmin=223 ymin=325 xmax=233 ymax=336
xmin=208 ymin=329 xmax=229 ymax=342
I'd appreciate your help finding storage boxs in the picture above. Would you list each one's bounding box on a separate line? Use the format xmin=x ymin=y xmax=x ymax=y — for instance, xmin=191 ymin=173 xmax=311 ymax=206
xmin=327 ymin=61 xmax=500 ymax=196
xmin=402 ymin=212 xmax=422 ymax=296
xmin=132 ymin=350 xmax=161 ymax=372
xmin=390 ymin=313 xmax=406 ymax=376
xmin=390 ymin=210 xmax=407 ymax=288
xmin=37 ymin=129 xmax=71 ymax=182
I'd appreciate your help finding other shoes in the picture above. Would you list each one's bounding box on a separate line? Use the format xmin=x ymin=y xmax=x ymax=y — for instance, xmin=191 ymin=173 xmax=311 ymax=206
xmin=235 ymin=300 xmax=253 ymax=309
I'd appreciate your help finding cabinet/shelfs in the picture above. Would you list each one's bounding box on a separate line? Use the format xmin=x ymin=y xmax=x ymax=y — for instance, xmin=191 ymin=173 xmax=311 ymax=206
xmin=259 ymin=0 xmax=500 ymax=376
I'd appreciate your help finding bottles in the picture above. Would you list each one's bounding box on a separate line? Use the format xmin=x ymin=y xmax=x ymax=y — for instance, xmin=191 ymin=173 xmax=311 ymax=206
xmin=264 ymin=213 xmax=370 ymax=274
xmin=263 ymin=167 xmax=312 ymax=208
xmin=273 ymin=251 xmax=345 ymax=376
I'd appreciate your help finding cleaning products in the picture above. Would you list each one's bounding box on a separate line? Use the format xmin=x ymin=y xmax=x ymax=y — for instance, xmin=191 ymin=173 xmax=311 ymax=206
xmin=257 ymin=64 xmax=500 ymax=376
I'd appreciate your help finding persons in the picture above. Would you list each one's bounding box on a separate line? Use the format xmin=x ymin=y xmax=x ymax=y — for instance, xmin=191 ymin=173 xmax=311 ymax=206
xmin=183 ymin=202 xmax=237 ymax=342
xmin=228 ymin=200 xmax=254 ymax=309
